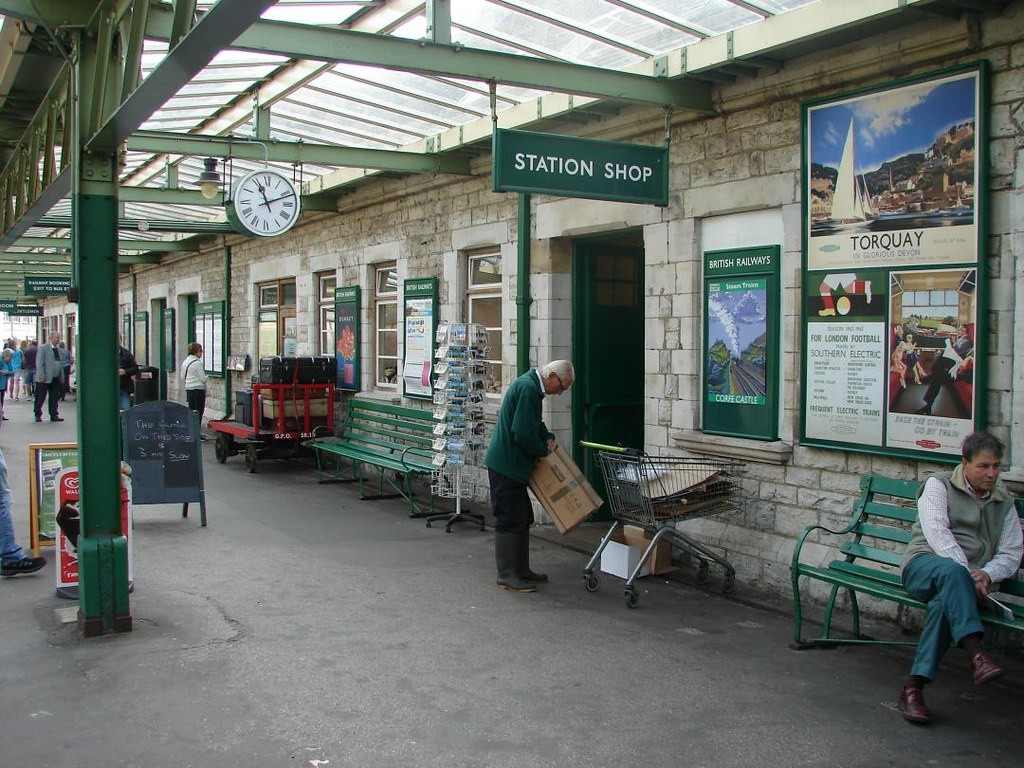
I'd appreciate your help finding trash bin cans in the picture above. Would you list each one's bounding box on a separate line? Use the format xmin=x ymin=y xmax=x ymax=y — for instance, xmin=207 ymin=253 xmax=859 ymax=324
xmin=133 ymin=365 xmax=158 ymax=405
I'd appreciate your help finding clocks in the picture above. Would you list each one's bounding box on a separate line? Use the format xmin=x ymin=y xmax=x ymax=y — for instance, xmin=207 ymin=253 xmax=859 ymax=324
xmin=225 ymin=169 xmax=302 ymax=239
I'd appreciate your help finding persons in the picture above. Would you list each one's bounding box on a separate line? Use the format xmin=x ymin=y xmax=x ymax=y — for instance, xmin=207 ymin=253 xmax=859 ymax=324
xmin=0 ymin=332 xmax=210 ymax=578
xmin=898 ymin=432 xmax=1024 ymax=723
xmin=487 ymin=360 xmax=575 ymax=592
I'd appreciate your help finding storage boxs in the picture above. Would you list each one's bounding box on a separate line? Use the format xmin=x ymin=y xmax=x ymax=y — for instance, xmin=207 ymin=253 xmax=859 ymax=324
xmin=527 ymin=441 xmax=604 ymax=536
xmin=600 ymin=536 xmax=640 ymax=579
xmin=623 ymin=525 xmax=671 ymax=575
xmin=235 ymin=355 xmax=336 ymax=429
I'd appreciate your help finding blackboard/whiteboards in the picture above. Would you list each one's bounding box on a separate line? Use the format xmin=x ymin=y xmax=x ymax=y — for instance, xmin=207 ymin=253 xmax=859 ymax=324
xmin=120 ymin=401 xmax=203 ymax=505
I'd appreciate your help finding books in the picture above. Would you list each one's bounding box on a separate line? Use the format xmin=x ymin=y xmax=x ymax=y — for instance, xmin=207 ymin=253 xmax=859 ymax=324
xmin=984 ymin=595 xmax=1015 ymax=621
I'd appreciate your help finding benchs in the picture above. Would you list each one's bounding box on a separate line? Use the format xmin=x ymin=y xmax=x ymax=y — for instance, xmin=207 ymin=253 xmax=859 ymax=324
xmin=300 ymin=397 xmax=471 ymax=518
xmin=788 ymin=476 xmax=1024 ymax=651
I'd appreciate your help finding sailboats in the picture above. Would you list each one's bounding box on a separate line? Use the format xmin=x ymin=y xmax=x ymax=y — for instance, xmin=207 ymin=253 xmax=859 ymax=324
xmin=815 ymin=117 xmax=877 ymax=231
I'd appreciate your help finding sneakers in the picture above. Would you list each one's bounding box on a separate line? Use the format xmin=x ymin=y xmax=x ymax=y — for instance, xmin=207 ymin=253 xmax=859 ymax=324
xmin=50 ymin=415 xmax=63 ymax=421
xmin=496 ymin=574 xmax=538 ymax=593
xmin=525 ymin=570 xmax=548 ymax=584
xmin=0 ymin=557 xmax=47 ymax=576
xmin=35 ymin=417 xmax=41 ymax=422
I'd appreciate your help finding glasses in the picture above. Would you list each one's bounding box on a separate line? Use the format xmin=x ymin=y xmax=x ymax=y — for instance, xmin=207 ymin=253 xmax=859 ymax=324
xmin=553 ymin=372 xmax=567 ymax=391
xmin=198 ymin=349 xmax=202 ymax=352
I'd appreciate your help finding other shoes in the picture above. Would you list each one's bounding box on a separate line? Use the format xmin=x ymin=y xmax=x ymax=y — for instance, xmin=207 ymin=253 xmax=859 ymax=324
xmin=2 ymin=414 xmax=8 ymax=420
xmin=26 ymin=396 xmax=32 ymax=401
xmin=10 ymin=395 xmax=13 ymax=397
xmin=15 ymin=397 xmax=18 ymax=401
xmin=200 ymin=437 xmax=205 ymax=441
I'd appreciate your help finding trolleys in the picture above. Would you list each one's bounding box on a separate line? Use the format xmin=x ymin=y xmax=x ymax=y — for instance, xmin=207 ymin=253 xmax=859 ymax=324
xmin=577 ymin=440 xmax=746 ymax=609
xmin=208 ymin=382 xmax=337 ymax=473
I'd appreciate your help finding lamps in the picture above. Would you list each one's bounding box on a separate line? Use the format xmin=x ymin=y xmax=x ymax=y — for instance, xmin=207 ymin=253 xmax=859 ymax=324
xmin=192 ymin=158 xmax=228 ymax=199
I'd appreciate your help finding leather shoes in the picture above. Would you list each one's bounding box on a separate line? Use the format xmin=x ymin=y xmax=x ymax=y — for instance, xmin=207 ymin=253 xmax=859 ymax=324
xmin=973 ymin=653 xmax=1002 ymax=687
xmin=898 ymin=689 xmax=932 ymax=721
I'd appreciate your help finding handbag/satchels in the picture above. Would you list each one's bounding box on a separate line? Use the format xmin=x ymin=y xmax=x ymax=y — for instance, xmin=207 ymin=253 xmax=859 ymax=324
xmin=70 ymin=354 xmax=74 ymax=364
